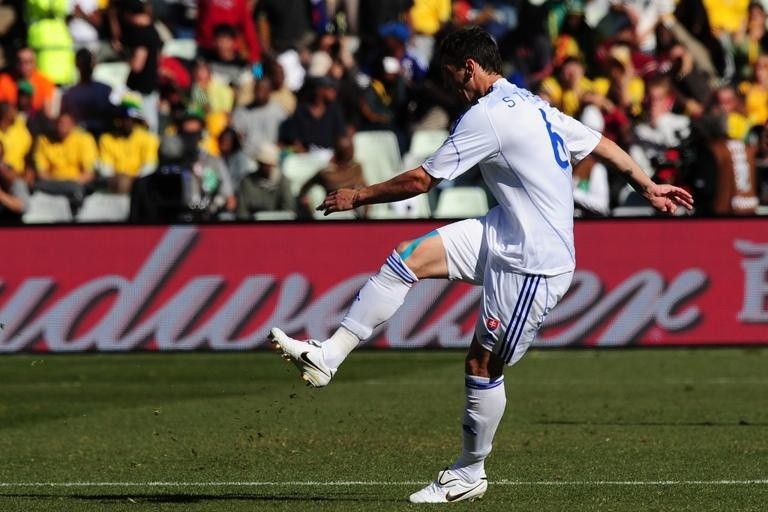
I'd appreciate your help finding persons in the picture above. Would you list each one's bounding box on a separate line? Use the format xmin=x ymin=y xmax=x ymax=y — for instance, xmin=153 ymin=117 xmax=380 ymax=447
xmin=266 ymin=25 xmax=699 ymax=506
xmin=0 ymin=1 xmax=767 ymax=228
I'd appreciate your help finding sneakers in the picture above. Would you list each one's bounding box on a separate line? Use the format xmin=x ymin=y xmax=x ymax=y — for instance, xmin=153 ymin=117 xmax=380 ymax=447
xmin=408 ymin=465 xmax=488 ymax=502
xmin=267 ymin=327 xmax=338 ymax=390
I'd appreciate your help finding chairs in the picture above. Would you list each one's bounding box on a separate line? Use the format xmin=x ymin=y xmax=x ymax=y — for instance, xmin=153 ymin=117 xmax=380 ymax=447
xmin=21 ymin=38 xmax=493 ymax=225
